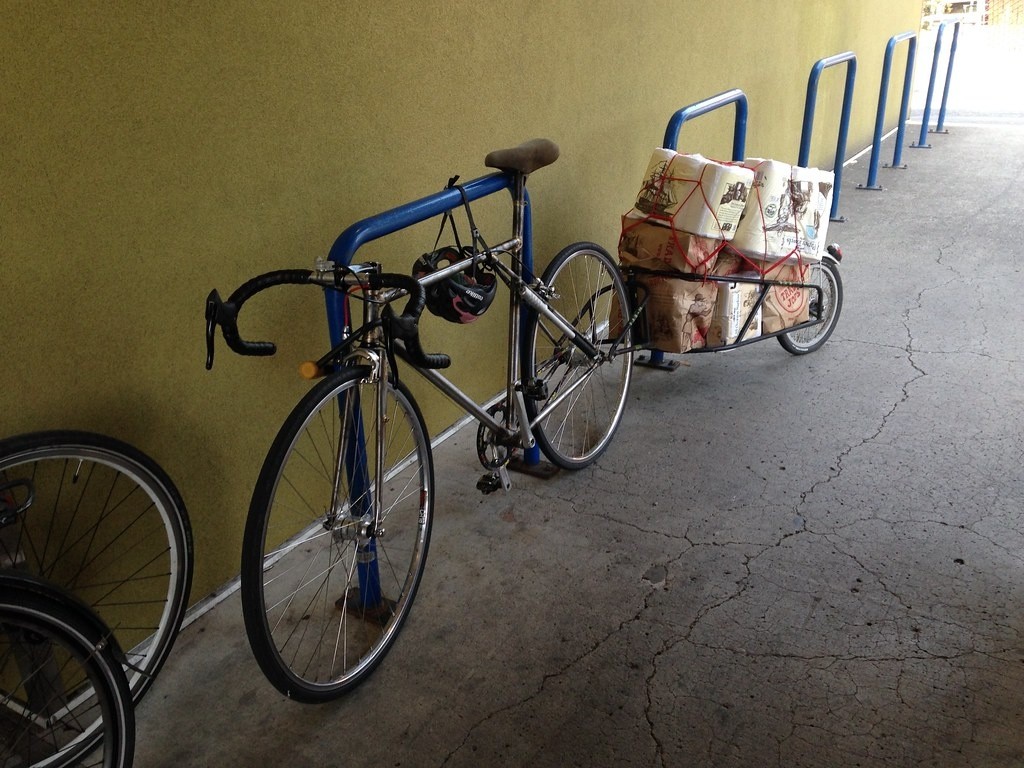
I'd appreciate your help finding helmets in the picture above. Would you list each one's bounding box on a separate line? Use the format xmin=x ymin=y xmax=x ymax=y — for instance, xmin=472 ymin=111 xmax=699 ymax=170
xmin=413 ymin=246 xmax=497 ymax=324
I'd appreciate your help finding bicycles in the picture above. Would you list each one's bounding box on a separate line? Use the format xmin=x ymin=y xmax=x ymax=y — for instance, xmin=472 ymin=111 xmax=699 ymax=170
xmin=1 ymin=427 xmax=195 ymax=768
xmin=205 ymin=139 xmax=635 ymax=704
